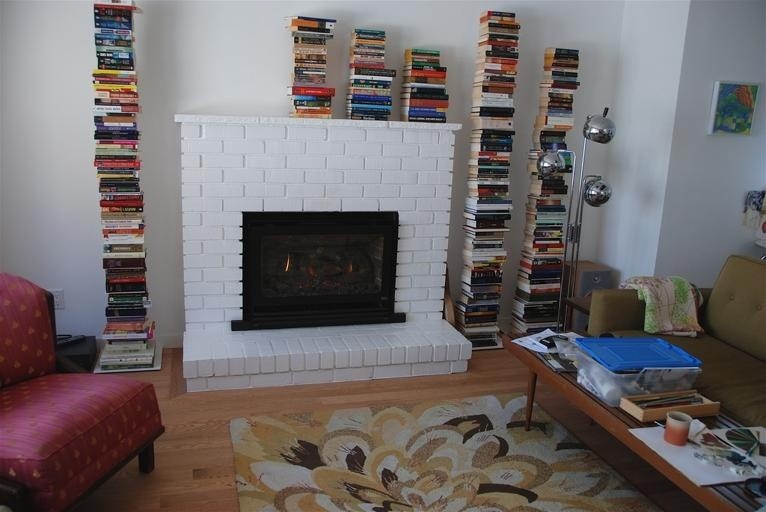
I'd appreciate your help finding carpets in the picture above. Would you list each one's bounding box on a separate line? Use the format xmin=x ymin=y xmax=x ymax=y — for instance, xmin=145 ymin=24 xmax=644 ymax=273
xmin=229 ymin=390 xmax=663 ymax=511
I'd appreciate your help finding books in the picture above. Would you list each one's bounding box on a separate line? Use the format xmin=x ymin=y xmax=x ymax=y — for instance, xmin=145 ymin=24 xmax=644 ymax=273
xmin=282 ymin=14 xmax=451 ymax=125
xmin=456 ymin=10 xmax=521 ymax=346
xmin=536 ymin=349 xmax=577 ymax=375
xmin=510 ymin=47 xmax=581 ymax=338
xmin=636 ymin=366 xmax=700 ymax=392
xmin=91 ymin=1 xmax=164 ymax=378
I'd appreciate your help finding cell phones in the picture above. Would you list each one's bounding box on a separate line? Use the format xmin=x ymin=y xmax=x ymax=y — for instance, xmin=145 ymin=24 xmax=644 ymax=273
xmin=540 ymin=335 xmax=568 ymax=348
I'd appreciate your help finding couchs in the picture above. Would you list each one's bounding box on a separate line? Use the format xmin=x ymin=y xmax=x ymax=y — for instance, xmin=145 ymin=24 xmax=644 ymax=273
xmin=588 ymin=253 xmax=766 ymax=427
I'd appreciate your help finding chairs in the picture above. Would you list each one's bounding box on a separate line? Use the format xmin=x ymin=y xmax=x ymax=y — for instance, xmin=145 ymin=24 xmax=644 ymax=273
xmin=0 ymin=273 xmax=166 ymax=512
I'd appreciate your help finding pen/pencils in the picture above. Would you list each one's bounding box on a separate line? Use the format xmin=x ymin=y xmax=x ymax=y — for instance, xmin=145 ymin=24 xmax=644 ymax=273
xmin=746 ymin=441 xmax=759 ymax=456
xmin=633 ymin=395 xmax=704 ymax=409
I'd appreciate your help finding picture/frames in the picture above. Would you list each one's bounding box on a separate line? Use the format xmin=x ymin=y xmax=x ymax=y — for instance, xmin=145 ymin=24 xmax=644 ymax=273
xmin=708 ymin=80 xmax=760 ymax=136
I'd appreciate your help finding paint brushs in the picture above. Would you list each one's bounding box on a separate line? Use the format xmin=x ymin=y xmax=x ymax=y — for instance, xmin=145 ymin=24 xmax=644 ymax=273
xmin=655 ymin=421 xmax=700 ymax=446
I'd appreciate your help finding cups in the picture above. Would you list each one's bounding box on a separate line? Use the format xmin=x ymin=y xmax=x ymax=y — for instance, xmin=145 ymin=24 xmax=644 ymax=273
xmin=663 ymin=410 xmax=693 ymax=447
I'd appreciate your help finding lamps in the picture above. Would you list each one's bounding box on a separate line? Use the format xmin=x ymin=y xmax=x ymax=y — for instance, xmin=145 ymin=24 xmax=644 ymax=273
xmin=537 ymin=108 xmax=616 ymax=334
